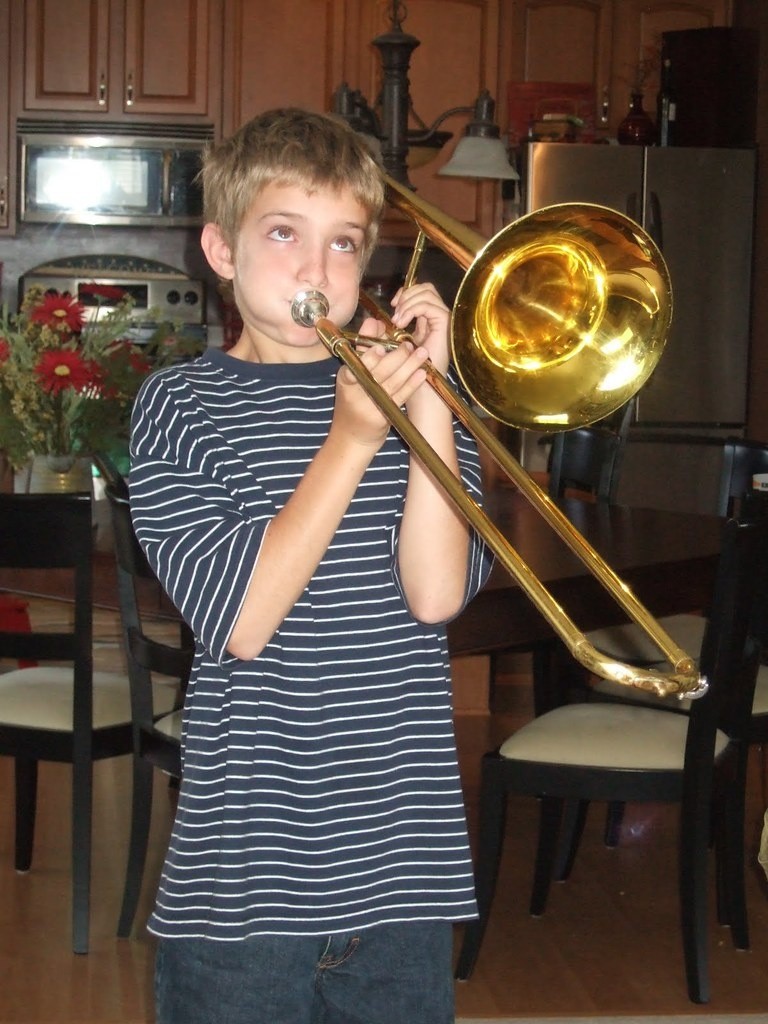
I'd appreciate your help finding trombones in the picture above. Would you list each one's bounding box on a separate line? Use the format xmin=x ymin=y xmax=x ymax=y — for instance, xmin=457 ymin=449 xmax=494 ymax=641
xmin=290 ymin=169 xmax=713 ymax=701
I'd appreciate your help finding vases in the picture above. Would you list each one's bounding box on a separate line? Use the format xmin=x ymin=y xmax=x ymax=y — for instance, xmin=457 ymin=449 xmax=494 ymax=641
xmin=12 ymin=453 xmax=114 ymax=554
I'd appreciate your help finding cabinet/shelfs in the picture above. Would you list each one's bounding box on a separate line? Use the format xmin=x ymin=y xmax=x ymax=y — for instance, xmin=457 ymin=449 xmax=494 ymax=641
xmin=0 ymin=0 xmax=18 ymax=239
xmin=496 ymin=1 xmax=736 ymax=145
xmin=213 ymin=0 xmax=500 ymax=250
xmin=18 ymin=1 xmax=217 ymax=128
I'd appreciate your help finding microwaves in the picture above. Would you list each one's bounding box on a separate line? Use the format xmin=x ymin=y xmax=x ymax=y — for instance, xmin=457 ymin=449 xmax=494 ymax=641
xmin=15 ymin=129 xmax=213 ymax=227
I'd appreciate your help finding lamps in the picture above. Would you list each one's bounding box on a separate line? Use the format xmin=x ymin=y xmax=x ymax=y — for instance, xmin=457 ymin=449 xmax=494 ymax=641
xmin=327 ymin=0 xmax=521 ymax=207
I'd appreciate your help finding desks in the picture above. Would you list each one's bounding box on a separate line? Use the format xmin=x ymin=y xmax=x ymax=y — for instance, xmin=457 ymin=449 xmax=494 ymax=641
xmin=0 ymin=493 xmax=728 ymax=886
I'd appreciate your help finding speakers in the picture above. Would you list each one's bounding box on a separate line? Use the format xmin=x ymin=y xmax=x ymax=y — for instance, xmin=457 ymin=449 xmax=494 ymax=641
xmin=661 ymin=26 xmax=760 ymax=149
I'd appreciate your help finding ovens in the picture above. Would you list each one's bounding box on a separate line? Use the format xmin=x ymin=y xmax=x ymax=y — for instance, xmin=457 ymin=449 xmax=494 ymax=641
xmin=17 ymin=274 xmax=206 ymax=369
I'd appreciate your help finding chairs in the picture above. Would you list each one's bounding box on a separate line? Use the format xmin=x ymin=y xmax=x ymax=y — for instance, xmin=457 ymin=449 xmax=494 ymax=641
xmin=104 ymin=482 xmax=194 ymax=937
xmin=0 ymin=491 xmax=177 ymax=956
xmin=456 ymin=494 xmax=767 ymax=1002
xmin=717 ymin=435 xmax=768 ymax=518
xmin=548 ymin=395 xmax=638 ymax=505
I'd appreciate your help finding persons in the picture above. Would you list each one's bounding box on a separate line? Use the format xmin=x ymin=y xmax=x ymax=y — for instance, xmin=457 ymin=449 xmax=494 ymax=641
xmin=127 ymin=106 xmax=496 ymax=1024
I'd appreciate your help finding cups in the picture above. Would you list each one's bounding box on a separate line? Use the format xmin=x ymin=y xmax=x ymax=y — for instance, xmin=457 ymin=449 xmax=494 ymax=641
xmin=752 ymin=473 xmax=768 ymax=491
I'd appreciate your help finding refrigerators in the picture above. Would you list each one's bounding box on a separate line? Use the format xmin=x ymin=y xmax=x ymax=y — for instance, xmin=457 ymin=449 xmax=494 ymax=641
xmin=521 ymin=141 xmax=758 ymax=435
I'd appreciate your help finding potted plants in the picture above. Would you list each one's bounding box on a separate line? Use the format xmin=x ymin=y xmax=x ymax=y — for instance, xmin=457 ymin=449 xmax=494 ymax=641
xmin=617 ymin=35 xmax=671 ymax=147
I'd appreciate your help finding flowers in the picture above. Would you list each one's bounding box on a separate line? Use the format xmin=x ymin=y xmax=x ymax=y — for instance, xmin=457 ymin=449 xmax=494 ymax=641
xmin=0 ymin=280 xmax=209 ymax=475
